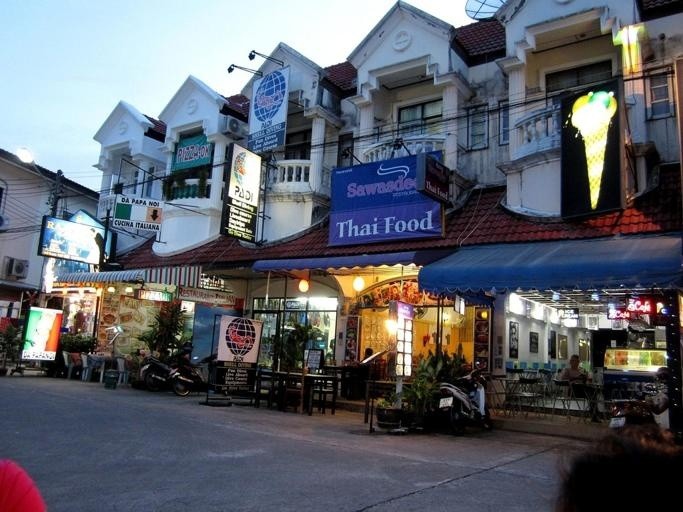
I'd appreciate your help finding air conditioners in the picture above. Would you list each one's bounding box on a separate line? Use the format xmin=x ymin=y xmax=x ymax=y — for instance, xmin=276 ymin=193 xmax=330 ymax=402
xmin=0 ymin=214 xmax=12 ymax=231
xmin=223 ymin=116 xmax=243 ymax=139
xmin=6 ymin=255 xmax=29 ymax=279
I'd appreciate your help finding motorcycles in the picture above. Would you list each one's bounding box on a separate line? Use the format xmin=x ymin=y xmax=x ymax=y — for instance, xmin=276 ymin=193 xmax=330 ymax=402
xmin=437 ymin=361 xmax=493 ymax=434
xmin=609 ymin=379 xmax=657 ymax=427
xmin=143 ymin=354 xmax=201 ymax=395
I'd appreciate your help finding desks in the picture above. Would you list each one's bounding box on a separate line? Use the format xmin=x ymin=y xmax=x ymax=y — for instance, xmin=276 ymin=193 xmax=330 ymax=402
xmin=255 ymin=367 xmax=338 ymax=415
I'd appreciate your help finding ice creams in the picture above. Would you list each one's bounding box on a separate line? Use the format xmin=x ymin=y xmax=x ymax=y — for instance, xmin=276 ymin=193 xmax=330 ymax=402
xmin=571 ymin=90 xmax=618 ymax=210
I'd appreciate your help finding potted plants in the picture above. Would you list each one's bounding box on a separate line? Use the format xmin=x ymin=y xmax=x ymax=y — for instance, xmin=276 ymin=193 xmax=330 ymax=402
xmin=0 ymin=324 xmax=35 ymax=376
xmin=375 ymin=399 xmax=402 ymax=428
xmin=401 ymin=386 xmax=417 ymax=427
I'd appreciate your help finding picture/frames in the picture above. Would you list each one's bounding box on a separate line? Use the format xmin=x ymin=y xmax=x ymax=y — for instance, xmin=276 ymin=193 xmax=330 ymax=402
xmin=558 ymin=333 xmax=567 ymax=360
xmin=509 ymin=321 xmax=519 ymax=359
xmin=529 ymin=332 xmax=538 ymax=353
xmin=579 ymin=339 xmax=590 ymax=361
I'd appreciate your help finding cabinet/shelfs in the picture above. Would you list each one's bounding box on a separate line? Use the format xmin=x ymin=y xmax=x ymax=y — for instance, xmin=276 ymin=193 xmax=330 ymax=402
xmin=472 ymin=306 xmax=492 ymax=373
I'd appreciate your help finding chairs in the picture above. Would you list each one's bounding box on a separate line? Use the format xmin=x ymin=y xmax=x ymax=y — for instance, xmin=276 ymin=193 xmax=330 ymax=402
xmin=62 ymin=349 xmax=137 ymax=386
xmin=485 ymin=367 xmax=628 ymax=426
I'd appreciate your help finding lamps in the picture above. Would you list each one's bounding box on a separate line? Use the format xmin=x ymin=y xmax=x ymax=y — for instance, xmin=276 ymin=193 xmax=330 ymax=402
xmin=339 ymin=146 xmax=365 ymax=165
xmin=353 ymin=276 xmax=364 ymax=291
xmin=108 ymin=287 xmax=115 ymax=292
xmin=393 ymin=137 xmax=413 ymax=157
xmin=227 ymin=63 xmax=263 ymax=78
xmin=299 ymin=279 xmax=308 ymax=292
xmin=125 ymin=286 xmax=133 ymax=292
xmin=248 ymin=49 xmax=284 ymax=68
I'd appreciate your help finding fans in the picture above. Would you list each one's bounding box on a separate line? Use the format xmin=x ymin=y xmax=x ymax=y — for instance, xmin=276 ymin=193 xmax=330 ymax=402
xmin=413 ymin=307 xmax=428 ymax=320
xmin=452 ymin=317 xmax=466 ymax=329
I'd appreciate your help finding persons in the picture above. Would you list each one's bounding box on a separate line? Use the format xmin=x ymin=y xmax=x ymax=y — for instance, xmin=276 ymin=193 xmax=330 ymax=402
xmin=361 ymin=348 xmax=373 ymax=362
xmin=555 ymin=424 xmax=683 ymax=511
xmin=560 ymin=354 xmax=602 ymax=424
